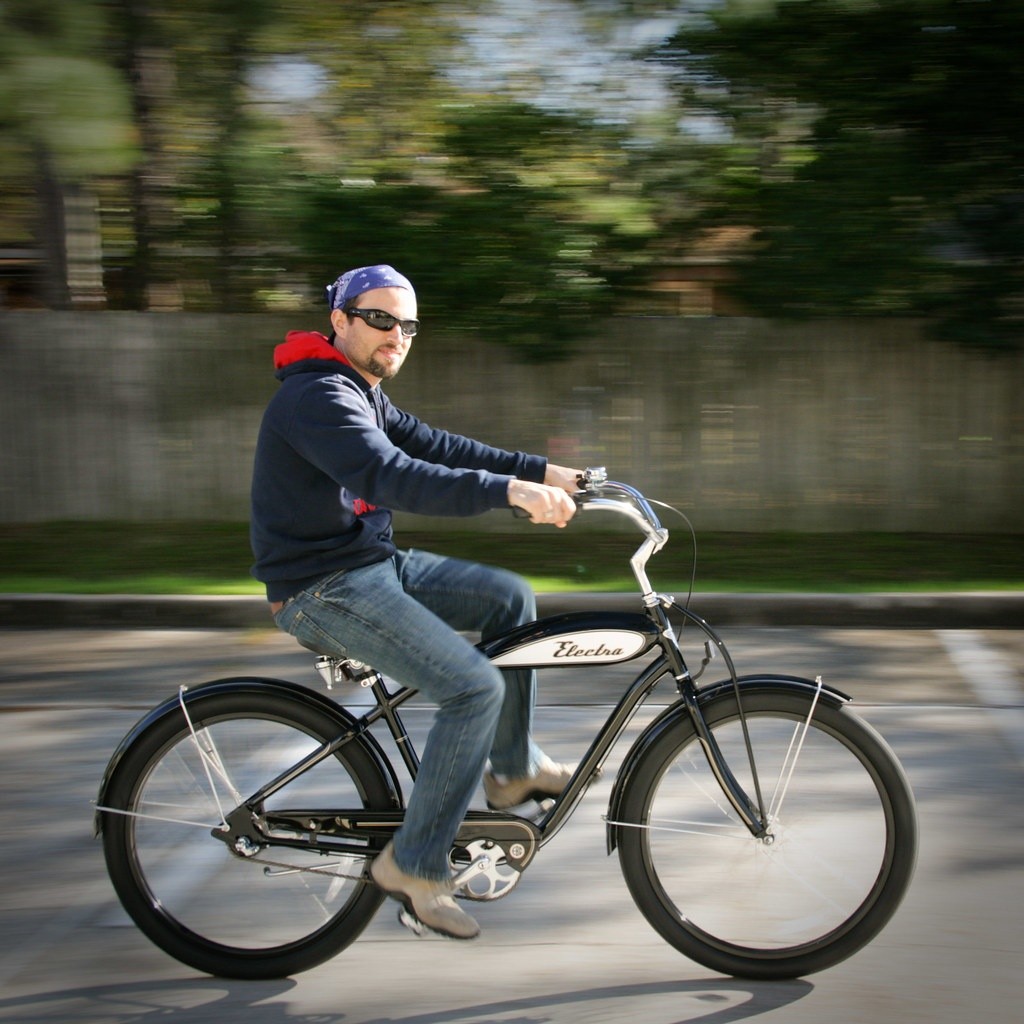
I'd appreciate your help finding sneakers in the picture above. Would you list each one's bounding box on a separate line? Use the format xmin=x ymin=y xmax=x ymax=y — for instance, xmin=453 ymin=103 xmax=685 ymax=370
xmin=482 ymin=750 xmax=605 ymax=811
xmin=371 ymin=838 xmax=481 ymax=940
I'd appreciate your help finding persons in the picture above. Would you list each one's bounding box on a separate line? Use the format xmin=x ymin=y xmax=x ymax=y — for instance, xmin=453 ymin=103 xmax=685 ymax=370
xmin=251 ymin=264 xmax=585 ymax=938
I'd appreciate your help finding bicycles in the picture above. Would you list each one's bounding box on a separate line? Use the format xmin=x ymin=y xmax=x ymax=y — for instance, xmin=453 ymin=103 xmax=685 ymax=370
xmin=92 ymin=468 xmax=918 ymax=984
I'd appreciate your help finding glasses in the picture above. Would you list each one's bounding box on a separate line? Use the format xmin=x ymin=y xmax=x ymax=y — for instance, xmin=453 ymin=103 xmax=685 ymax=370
xmin=346 ymin=305 xmax=422 ymax=338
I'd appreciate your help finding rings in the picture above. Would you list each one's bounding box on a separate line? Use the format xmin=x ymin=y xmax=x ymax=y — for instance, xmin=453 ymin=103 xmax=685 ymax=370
xmin=545 ymin=509 xmax=556 ymax=517
